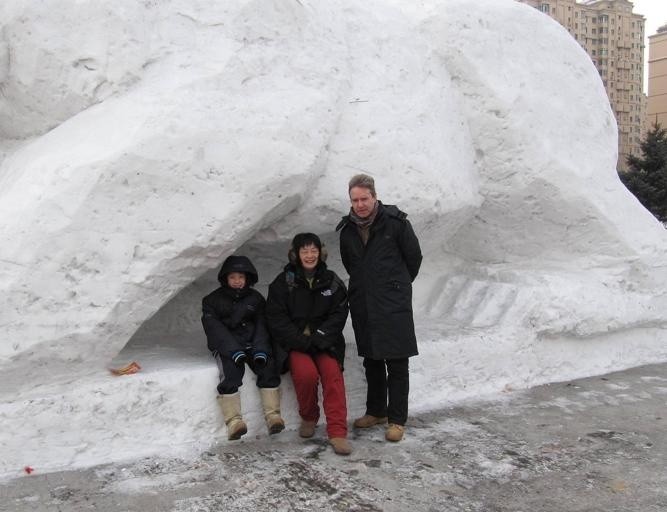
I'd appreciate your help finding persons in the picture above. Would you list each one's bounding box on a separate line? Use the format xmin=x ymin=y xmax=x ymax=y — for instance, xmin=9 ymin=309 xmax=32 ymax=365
xmin=335 ymin=173 xmax=422 ymax=444
xmin=200 ymin=253 xmax=285 ymax=440
xmin=263 ymin=231 xmax=352 ymax=456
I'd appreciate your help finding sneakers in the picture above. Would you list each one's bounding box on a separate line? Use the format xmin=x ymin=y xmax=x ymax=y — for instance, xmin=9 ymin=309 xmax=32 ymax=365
xmin=354 ymin=413 xmax=388 ymax=429
xmin=329 ymin=437 xmax=353 ymax=455
xmin=300 ymin=418 xmax=320 ymax=438
xmin=385 ymin=423 xmax=404 ymax=441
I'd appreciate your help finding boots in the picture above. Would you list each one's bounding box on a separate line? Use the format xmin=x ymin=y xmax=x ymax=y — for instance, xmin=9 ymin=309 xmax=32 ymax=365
xmin=216 ymin=391 xmax=247 ymax=441
xmin=258 ymin=387 xmax=285 ymax=436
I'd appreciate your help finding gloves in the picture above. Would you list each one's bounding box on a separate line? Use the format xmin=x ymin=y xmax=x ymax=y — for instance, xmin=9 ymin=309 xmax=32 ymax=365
xmin=252 ymin=352 xmax=267 ymax=368
xmin=232 ymin=352 xmax=248 ymax=368
xmin=295 ymin=334 xmax=316 ymax=356
xmin=313 ymin=329 xmax=334 ymax=351
